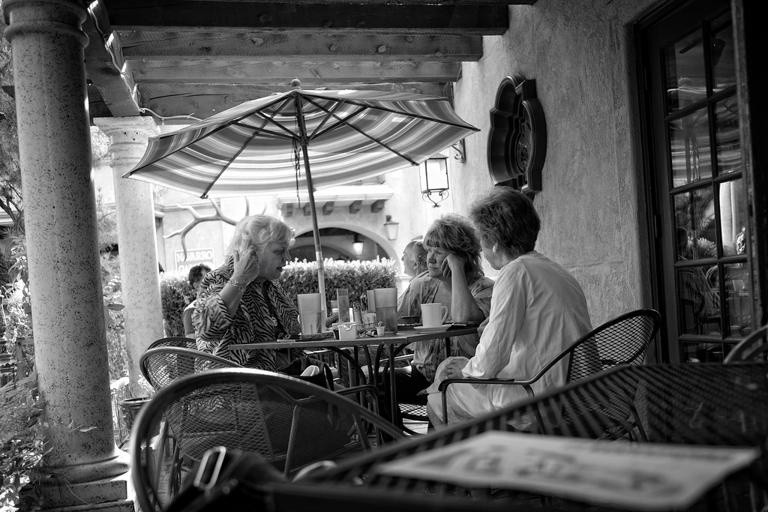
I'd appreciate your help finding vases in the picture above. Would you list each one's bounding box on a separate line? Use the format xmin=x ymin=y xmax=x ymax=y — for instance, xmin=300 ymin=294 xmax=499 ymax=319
xmin=117 ymin=396 xmax=160 ymax=440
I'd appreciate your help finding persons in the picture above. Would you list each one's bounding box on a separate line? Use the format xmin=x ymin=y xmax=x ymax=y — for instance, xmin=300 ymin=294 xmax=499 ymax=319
xmin=394 ymin=238 xmax=429 ymax=321
xmin=364 ymin=213 xmax=495 ymax=443
xmin=182 ymin=263 xmax=211 ymax=348
xmin=424 ymin=185 xmax=604 ymax=433
xmin=189 ymin=213 xmax=350 ymax=467
xmin=674 ymin=227 xmax=715 ymax=336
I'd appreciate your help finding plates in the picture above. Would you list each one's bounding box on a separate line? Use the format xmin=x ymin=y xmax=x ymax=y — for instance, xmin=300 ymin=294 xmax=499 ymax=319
xmin=413 ymin=325 xmax=451 ymax=333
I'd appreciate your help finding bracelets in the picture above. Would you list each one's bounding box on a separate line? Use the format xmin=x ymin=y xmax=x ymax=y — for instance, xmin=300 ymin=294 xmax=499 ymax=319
xmin=227 ymin=278 xmax=247 ymax=294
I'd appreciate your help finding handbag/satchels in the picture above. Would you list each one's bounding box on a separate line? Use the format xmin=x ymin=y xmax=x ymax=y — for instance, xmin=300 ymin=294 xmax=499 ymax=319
xmin=273 ymin=355 xmax=339 ymax=431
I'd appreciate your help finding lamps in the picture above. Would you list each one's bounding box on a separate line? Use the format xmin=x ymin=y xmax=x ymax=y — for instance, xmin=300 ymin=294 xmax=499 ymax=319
xmin=352 ymin=232 xmax=364 ymax=259
xmin=384 ymin=214 xmax=399 ymax=241
xmin=420 ymin=138 xmax=465 ymax=209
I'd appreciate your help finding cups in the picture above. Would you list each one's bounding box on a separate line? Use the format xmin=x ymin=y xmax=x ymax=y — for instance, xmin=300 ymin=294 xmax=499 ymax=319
xmin=420 ymin=302 xmax=448 ymax=328
xmin=297 ymin=287 xmax=399 ymax=341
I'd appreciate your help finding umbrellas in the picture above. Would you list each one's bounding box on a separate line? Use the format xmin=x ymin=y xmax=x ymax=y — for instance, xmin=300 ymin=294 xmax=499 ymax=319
xmin=120 ymin=75 xmax=482 ymax=319
